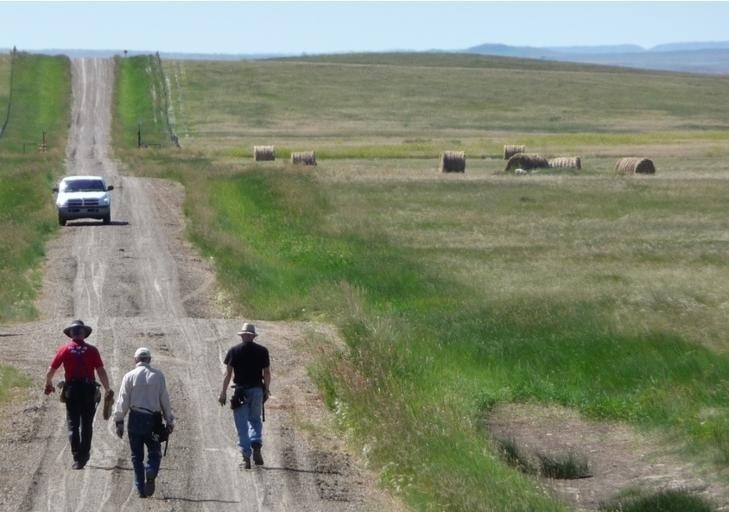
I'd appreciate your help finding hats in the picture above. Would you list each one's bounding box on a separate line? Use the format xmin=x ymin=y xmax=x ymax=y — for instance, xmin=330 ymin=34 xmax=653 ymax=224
xmin=236 ymin=322 xmax=259 ymax=337
xmin=63 ymin=319 xmax=92 ymax=340
xmin=134 ymin=346 xmax=153 ymax=359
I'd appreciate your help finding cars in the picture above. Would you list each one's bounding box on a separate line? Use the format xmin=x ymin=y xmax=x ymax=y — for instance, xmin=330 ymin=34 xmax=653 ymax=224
xmin=53 ymin=176 xmax=114 ymax=226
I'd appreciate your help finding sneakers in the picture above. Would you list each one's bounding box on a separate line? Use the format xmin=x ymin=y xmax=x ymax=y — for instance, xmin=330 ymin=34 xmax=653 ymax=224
xmin=252 ymin=443 xmax=264 ymax=466
xmin=242 ymin=457 xmax=251 ymax=469
xmin=136 ymin=477 xmax=156 ymax=498
xmin=72 ymin=452 xmax=90 ymax=470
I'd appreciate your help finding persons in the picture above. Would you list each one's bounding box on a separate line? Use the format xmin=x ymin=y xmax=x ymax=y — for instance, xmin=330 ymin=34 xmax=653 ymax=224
xmin=216 ymin=320 xmax=272 ymax=471
xmin=40 ymin=316 xmax=112 ymax=468
xmin=112 ymin=346 xmax=172 ymax=499
